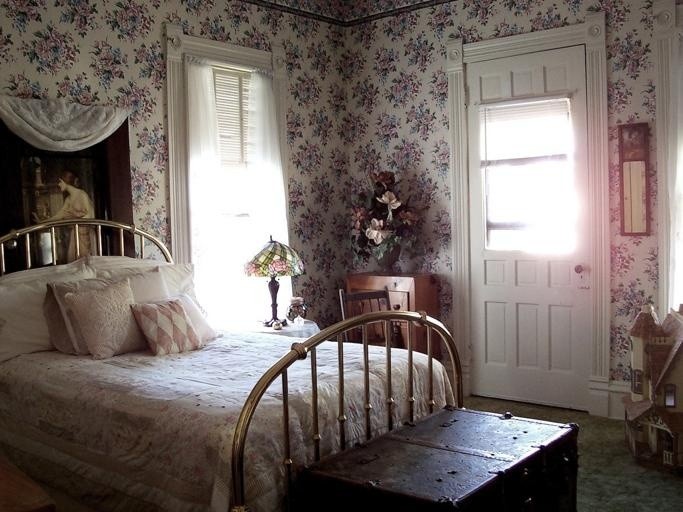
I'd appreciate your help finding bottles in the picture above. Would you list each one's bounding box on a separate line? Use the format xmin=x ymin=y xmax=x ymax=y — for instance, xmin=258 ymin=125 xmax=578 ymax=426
xmin=287 ymin=296 xmax=307 ymax=322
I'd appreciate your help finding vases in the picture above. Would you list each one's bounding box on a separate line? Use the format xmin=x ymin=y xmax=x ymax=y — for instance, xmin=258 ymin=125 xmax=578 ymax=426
xmin=375 ymin=244 xmax=402 ymax=276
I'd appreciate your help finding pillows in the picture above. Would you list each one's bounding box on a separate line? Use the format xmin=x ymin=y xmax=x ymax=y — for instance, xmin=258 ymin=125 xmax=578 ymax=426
xmin=0 ymin=255 xmax=219 ymax=360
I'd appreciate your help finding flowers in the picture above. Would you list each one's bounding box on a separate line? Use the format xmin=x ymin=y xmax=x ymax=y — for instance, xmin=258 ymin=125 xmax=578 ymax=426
xmin=346 ymin=170 xmax=424 ymax=267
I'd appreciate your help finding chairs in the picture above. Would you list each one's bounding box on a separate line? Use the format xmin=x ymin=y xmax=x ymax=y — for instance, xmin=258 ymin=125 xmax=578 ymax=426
xmin=338 ymin=288 xmax=399 ymax=348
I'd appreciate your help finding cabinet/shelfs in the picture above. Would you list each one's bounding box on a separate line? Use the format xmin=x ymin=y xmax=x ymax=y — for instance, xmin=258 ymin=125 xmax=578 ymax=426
xmin=343 ymin=273 xmax=441 ymax=362
xmin=310 ymin=408 xmax=580 ymax=512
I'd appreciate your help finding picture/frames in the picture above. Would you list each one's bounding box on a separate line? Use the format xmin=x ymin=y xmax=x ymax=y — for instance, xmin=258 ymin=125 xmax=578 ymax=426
xmin=0 ymin=96 xmax=135 ymax=274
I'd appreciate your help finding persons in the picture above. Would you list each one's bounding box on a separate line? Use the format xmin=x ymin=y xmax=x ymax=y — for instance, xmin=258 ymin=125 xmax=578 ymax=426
xmin=31 ymin=171 xmax=97 ymax=264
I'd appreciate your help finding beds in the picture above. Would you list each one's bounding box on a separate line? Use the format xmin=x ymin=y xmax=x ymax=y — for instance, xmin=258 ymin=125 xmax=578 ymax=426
xmin=0 ymin=218 xmax=465 ymax=512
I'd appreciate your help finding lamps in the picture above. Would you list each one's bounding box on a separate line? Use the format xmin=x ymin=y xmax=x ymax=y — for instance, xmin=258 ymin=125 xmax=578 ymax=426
xmin=245 ymin=236 xmax=305 ymax=328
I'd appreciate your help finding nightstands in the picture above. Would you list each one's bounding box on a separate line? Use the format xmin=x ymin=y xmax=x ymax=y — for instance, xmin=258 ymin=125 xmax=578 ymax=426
xmin=255 ymin=316 xmax=321 ymax=337
